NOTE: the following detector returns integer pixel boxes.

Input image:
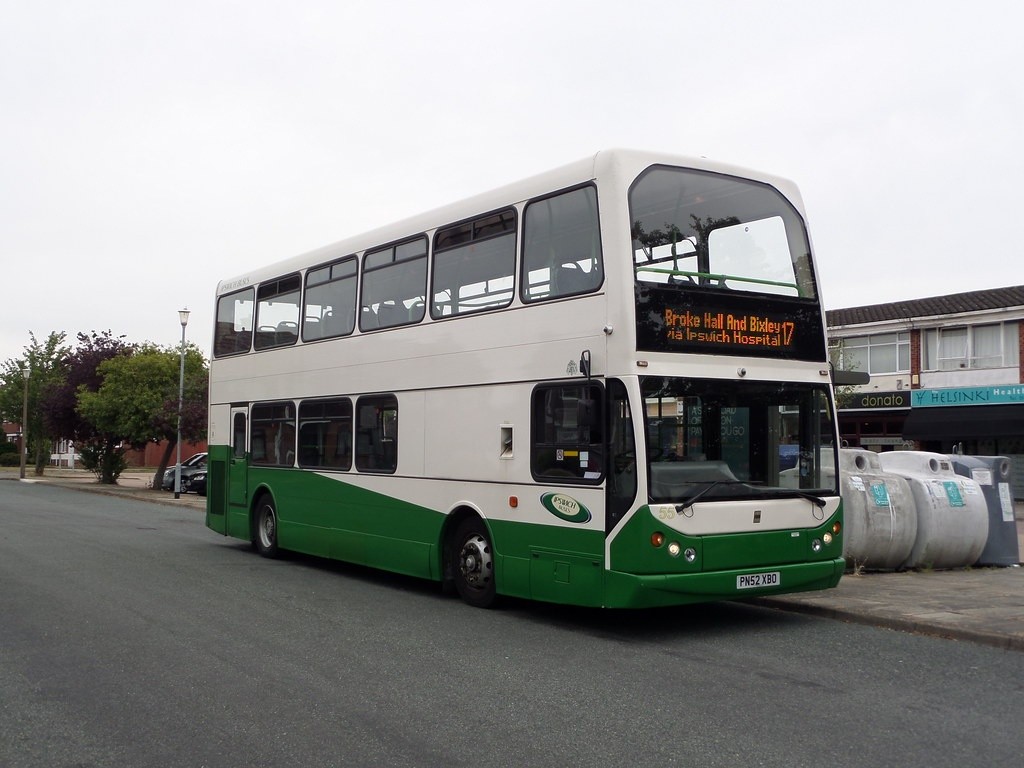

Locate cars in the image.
[176,453,209,496]
[155,452,208,491]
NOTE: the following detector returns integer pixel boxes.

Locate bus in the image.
[206,148,846,614]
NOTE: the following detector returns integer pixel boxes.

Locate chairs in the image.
[218,255,731,357]
